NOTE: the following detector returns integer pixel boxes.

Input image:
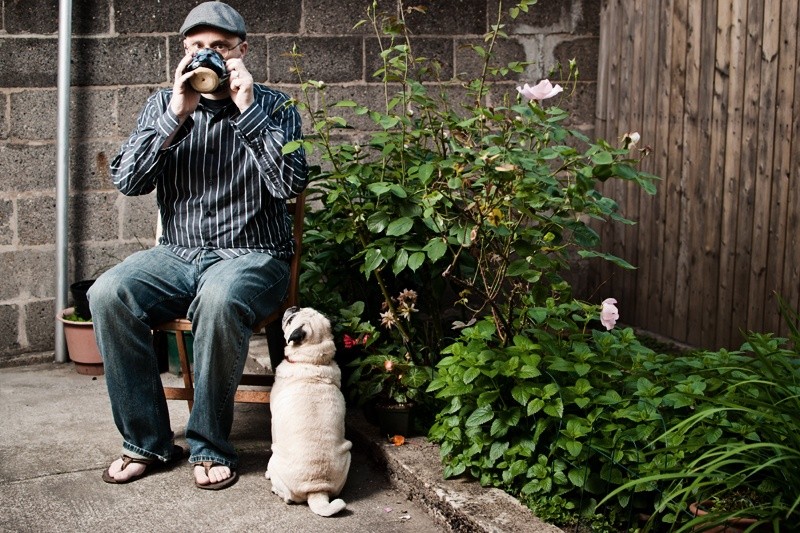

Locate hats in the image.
[180,1,247,41]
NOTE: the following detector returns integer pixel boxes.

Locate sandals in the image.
[192,461,237,490]
[102,432,183,484]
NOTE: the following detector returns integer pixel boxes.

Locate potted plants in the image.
[54,306,104,378]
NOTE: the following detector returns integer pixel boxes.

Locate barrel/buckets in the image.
[70,279,96,320]
[166,330,194,374]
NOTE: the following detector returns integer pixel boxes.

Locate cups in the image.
[186,48,231,92]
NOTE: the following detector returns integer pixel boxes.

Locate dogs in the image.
[266,304,353,518]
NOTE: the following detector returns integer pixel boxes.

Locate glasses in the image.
[185,41,244,57]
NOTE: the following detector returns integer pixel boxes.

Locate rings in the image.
[232,70,240,78]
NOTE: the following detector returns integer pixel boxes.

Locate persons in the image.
[86,0,310,491]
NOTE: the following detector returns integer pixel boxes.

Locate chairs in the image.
[151,161,323,420]
